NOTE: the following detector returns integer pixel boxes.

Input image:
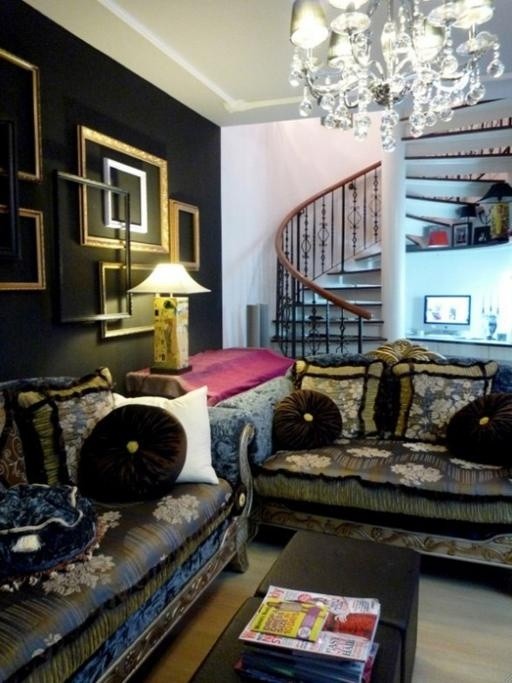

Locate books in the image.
[233,583,382,682]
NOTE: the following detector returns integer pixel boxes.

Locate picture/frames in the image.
[451,222,471,248]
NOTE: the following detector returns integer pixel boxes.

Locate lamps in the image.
[477,179,512,238]
[287,0,505,153]
[127,261,212,375]
